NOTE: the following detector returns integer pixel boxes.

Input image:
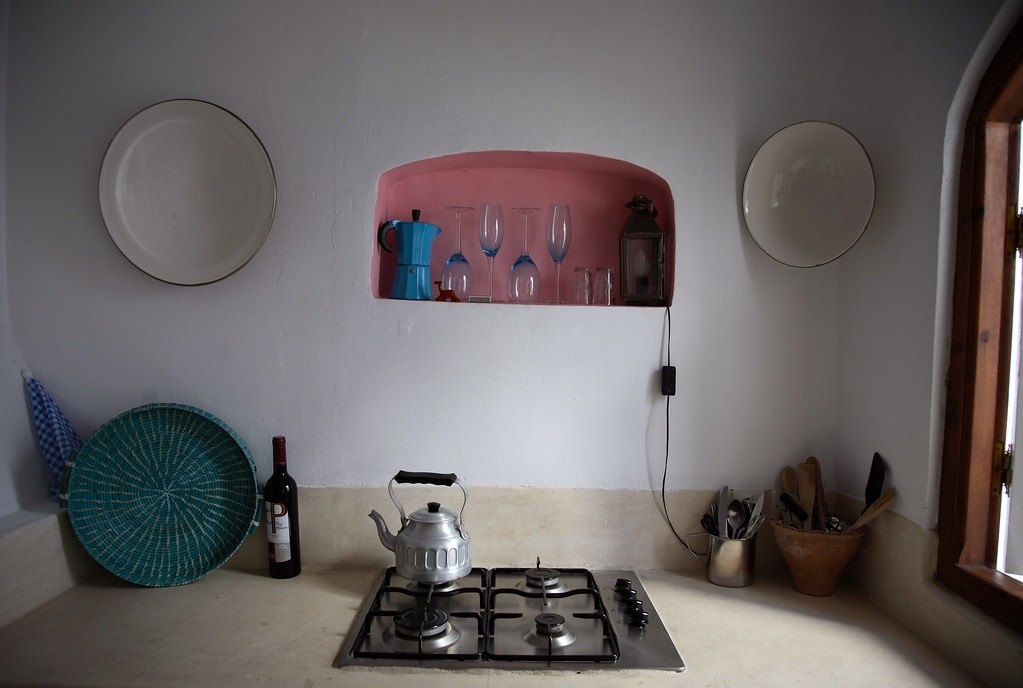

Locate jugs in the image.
[378,208,442,301]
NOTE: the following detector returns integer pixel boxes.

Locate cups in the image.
[686,532,757,588]
[575,267,615,305]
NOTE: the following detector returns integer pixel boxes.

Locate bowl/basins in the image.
[741,121,877,269]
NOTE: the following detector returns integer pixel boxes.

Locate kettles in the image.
[368,470,472,586]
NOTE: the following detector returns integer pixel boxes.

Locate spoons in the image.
[726,499,756,539]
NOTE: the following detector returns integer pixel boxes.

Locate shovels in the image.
[799,462,816,531]
[861,451,885,516]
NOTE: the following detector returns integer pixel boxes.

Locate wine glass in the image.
[441,203,572,304]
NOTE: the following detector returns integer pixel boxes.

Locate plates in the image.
[97,99,279,287]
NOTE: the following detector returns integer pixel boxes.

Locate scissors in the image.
[701,513,718,537]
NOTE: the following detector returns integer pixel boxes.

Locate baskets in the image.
[67,403,265,587]
[768,520,867,596]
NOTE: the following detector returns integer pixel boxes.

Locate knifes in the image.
[740,491,767,540]
[718,485,733,540]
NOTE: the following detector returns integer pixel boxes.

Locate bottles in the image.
[263,436,303,580]
[619,193,666,306]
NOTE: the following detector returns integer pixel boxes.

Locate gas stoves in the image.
[330,557,686,673]
